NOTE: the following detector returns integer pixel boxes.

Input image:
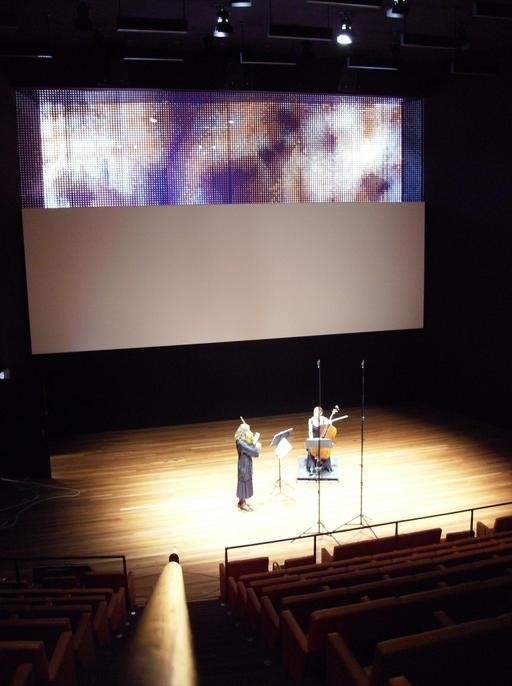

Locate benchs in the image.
[0,565,135,686]
[220,515,512,686]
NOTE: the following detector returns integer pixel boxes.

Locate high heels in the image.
[238,503,253,512]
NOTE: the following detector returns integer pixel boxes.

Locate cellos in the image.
[308,404,340,460]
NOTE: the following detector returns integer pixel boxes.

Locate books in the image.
[275,438,293,459]
[305,438,333,448]
[270,427,294,447]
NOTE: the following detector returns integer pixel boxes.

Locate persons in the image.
[304,406,334,476]
[234,423,261,513]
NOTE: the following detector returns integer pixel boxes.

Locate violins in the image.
[238,416,255,445]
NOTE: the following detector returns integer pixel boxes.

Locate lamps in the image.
[169,553,179,563]
[336,5,353,45]
[213,0,233,37]
[386,0,407,18]
